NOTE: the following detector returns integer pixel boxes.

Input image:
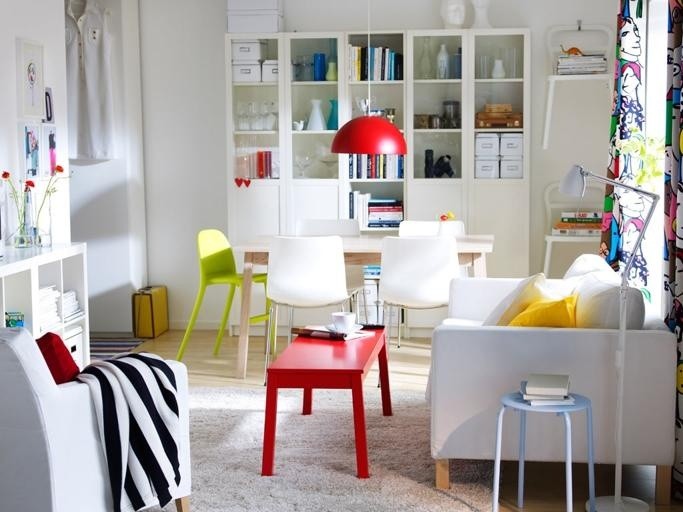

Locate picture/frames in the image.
[13,34,46,123]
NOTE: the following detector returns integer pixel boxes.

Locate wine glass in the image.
[295,145,338,179]
[356,97,396,126]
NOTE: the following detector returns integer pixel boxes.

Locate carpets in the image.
[89,336,145,365]
[138,385,501,511]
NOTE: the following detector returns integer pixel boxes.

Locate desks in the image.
[256,325,393,480]
[235,233,496,383]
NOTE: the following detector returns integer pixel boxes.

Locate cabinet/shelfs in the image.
[223,31,348,341]
[0,241,91,373]
[408,26,533,344]
[347,28,405,326]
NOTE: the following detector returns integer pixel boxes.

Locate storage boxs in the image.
[475,131,522,177]
[226,13,281,33]
[229,36,279,85]
[226,0,282,15]
[60,326,84,371]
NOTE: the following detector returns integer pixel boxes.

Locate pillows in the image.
[497,273,545,326]
[508,289,577,327]
[34,332,79,383]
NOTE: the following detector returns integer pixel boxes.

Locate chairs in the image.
[394,219,472,348]
[263,234,363,387]
[424,252,677,506]
[173,227,275,363]
[377,234,457,386]
[0,325,193,511]
[296,221,367,325]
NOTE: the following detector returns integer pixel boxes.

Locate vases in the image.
[303,98,327,130]
[324,98,339,131]
[11,227,39,247]
[434,42,450,80]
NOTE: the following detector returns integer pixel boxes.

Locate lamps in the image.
[558,163,659,509]
[327,2,408,155]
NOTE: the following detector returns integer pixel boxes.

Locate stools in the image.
[490,392,597,511]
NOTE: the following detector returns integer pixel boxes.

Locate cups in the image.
[479,45,519,80]
[332,311,355,328]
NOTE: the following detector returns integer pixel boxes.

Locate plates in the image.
[326,324,363,334]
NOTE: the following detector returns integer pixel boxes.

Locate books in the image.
[551,209,602,236]
[39,284,83,330]
[349,154,403,229]
[557,55,608,75]
[290,325,375,342]
[348,43,401,80]
[520,373,575,407]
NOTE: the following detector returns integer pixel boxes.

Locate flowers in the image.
[1,163,70,239]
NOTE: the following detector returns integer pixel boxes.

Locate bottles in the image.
[291,54,337,130]
[235,100,279,178]
[439,0,466,29]
[430,44,461,128]
[425,149,433,178]
